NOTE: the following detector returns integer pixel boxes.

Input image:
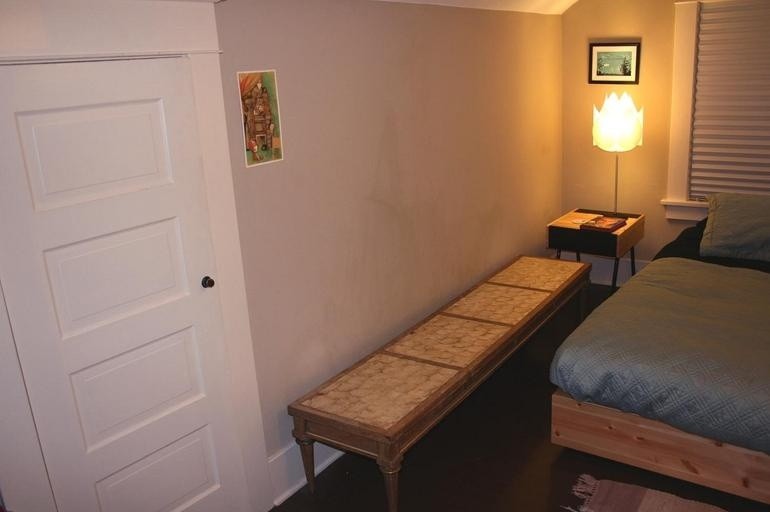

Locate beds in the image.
[550,216,770,505]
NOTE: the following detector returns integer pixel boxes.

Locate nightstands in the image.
[547,208,646,293]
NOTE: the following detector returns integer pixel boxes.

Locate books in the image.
[579,215,628,234]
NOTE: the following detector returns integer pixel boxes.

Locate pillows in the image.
[701,192,770,262]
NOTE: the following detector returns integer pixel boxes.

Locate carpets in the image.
[559,474,728,512]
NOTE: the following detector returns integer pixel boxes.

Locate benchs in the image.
[287,255,592,512]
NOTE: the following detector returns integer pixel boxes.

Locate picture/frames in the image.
[588,41,641,84]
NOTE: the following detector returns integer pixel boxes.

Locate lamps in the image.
[593,91,644,213]
